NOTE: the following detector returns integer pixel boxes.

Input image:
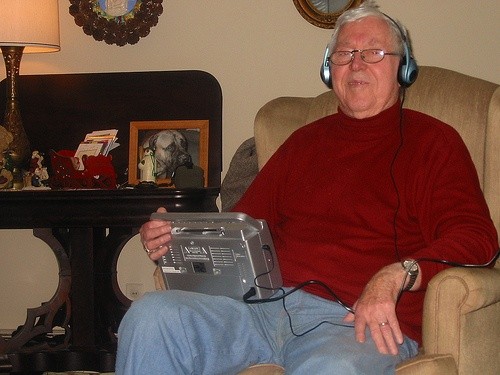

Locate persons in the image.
[115,6,499,375]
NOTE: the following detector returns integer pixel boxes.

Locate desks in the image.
[0,70,223,375]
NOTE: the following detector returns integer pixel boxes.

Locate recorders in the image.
[149,212,283,301]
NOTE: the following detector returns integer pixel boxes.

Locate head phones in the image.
[320,15,419,90]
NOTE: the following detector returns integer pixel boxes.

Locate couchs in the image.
[153,66,500,375]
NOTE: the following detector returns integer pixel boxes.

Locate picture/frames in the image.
[128,120,209,188]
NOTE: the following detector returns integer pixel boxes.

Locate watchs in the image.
[400,258,418,292]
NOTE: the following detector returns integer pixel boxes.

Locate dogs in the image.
[140,129,192,180]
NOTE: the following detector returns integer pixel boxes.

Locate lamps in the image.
[0,0,60,189]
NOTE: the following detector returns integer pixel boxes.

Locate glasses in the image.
[328,49,402,66]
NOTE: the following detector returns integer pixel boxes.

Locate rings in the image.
[379,322,388,326]
[143,243,152,253]
